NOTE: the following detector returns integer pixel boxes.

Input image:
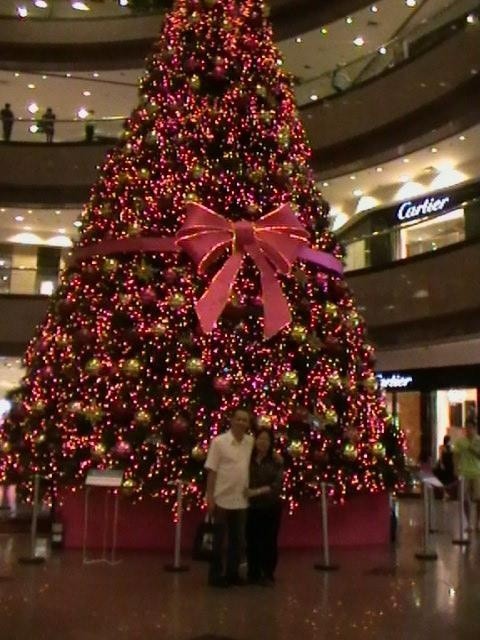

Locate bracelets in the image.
[257,487,261,496]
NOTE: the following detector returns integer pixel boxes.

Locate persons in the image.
[242,426,286,585]
[41,106,56,145]
[433,435,456,501]
[0,103,16,142]
[84,108,97,145]
[202,407,286,587]
[454,533,480,640]
[451,418,480,534]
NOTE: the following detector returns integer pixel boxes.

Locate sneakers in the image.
[209,572,250,589]
